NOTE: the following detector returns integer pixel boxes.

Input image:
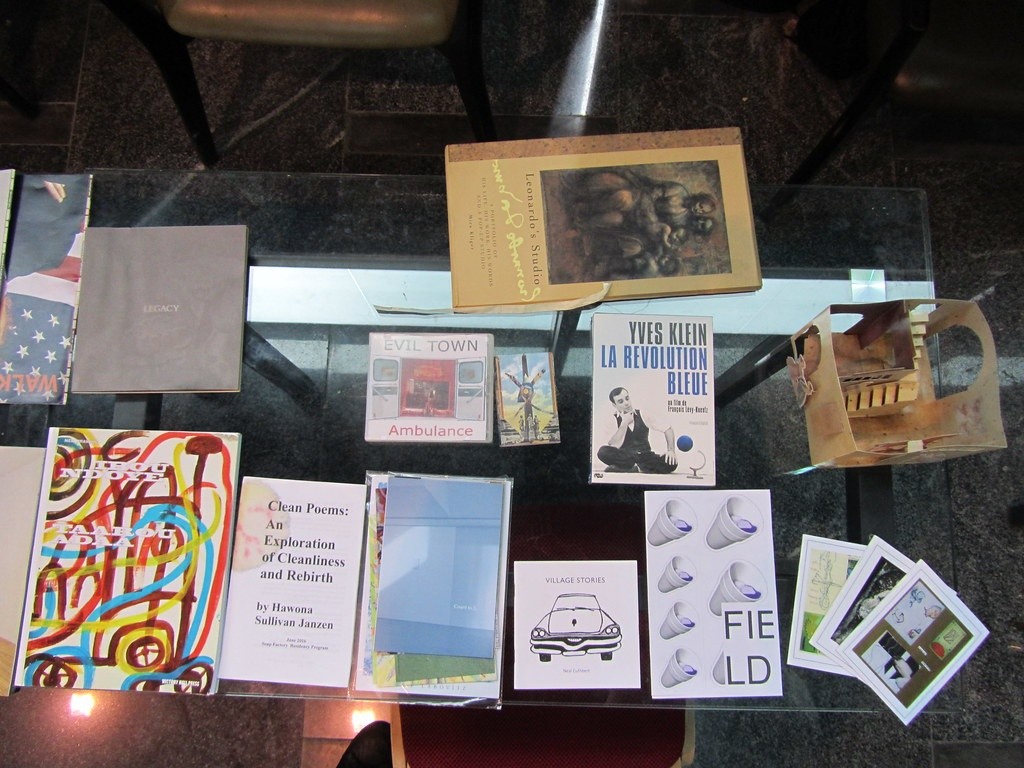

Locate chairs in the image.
[100,0,498,170]
[335,503,695,768]
[759,0,1024,224]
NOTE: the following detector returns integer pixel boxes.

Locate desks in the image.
[0,176,971,720]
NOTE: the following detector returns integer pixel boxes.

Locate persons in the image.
[597,387,678,474]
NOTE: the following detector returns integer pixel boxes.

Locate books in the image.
[786,534,990,726]
[0,426,513,707]
[0,169,248,407]
[444,125,762,315]
[587,313,715,488]
[365,333,561,447]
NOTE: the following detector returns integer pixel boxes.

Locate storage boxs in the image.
[789,298,1009,471]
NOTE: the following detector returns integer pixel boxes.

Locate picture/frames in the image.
[835,558,992,726]
[784,534,867,679]
[807,534,917,687]
